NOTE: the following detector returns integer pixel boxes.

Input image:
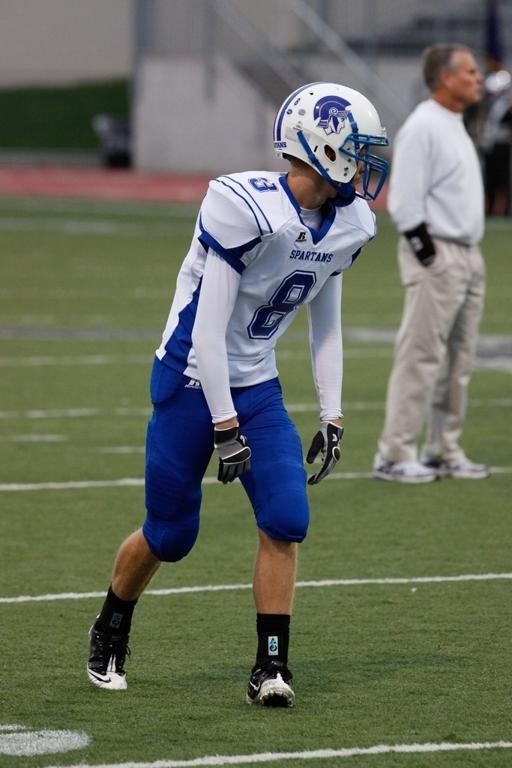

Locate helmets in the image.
[272,81,382,184]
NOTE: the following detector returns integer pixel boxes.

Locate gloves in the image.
[214,424,252,484]
[305,422,344,485]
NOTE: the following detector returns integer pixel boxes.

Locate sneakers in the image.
[246,665,296,707]
[87,613,128,690]
[372,450,438,483]
[422,446,489,479]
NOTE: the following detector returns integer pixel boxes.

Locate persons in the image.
[86,82,390,706]
[369,41,489,486]
